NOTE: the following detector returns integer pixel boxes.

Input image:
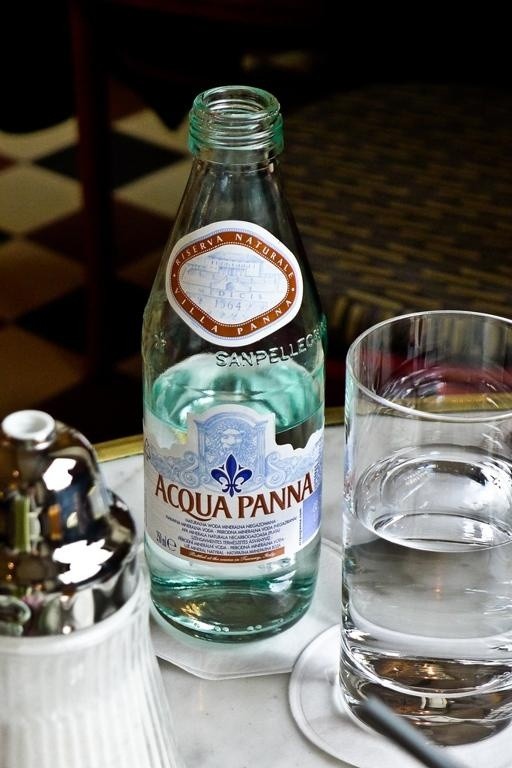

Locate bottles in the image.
[140,81,327,642]
[1,401,184,767]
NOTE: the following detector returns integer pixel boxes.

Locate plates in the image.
[290,620,510,768]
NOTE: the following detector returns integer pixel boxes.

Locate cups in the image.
[337,306,512,744]
